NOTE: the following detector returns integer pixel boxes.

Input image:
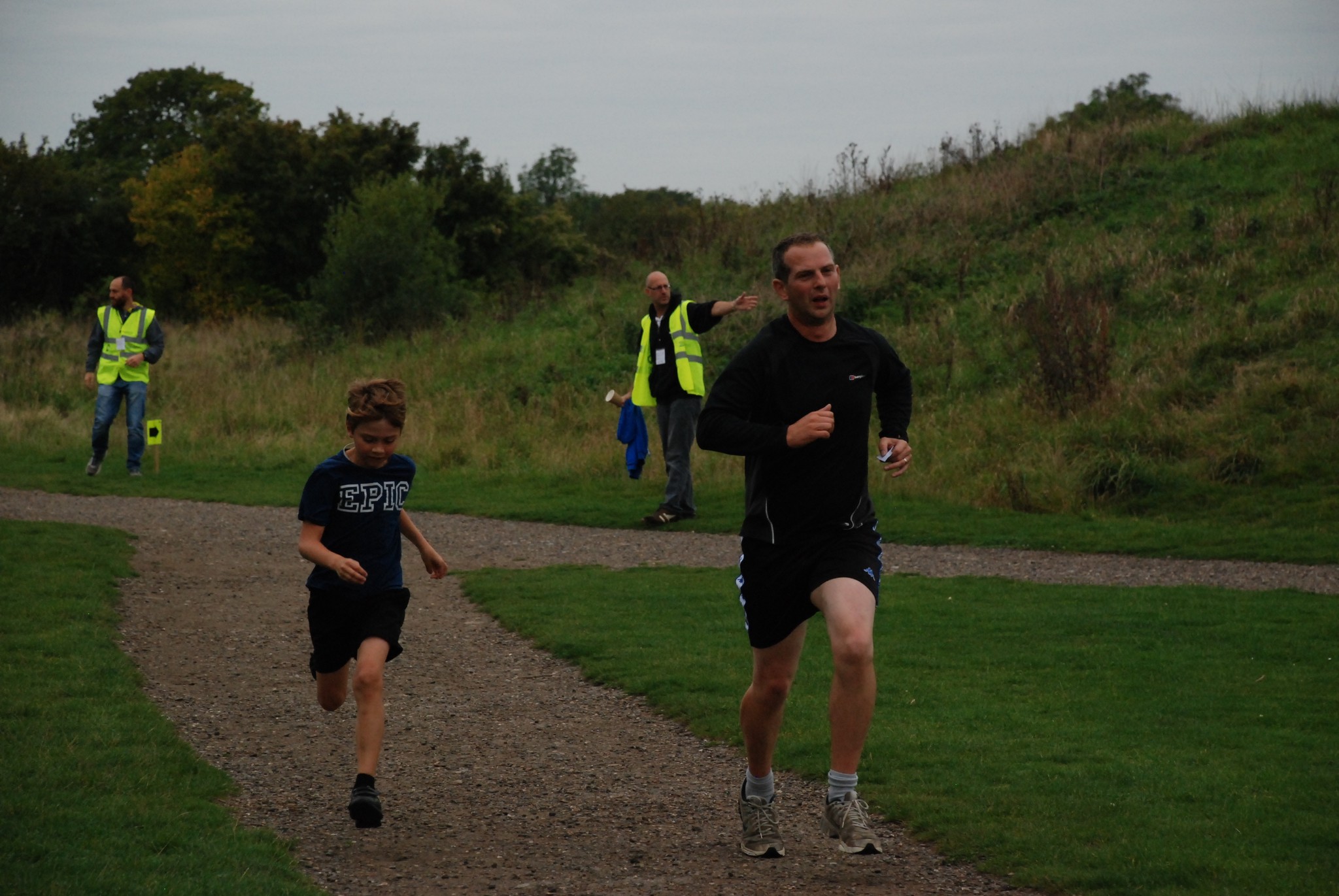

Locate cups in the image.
[605,389,624,407]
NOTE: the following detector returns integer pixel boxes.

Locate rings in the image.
[904,458,907,464]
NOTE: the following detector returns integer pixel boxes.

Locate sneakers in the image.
[309,652,316,679]
[738,776,785,857]
[86,458,101,475]
[130,471,141,477]
[348,786,383,828]
[820,790,882,855]
[644,509,691,525]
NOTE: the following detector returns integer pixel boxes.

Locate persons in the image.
[296,377,449,829]
[695,232,913,859]
[614,271,758,524]
[85,277,166,476]
